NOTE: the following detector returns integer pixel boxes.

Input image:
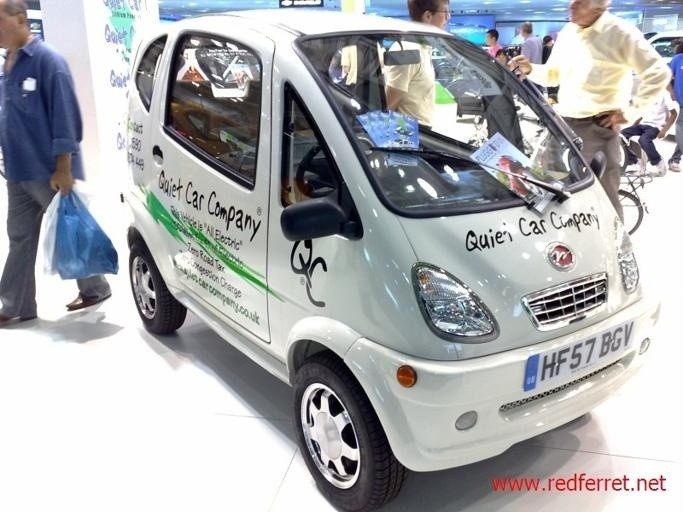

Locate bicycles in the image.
[619,175,653,235]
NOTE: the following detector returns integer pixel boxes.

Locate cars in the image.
[120,7,661,512]
[646,31,683,63]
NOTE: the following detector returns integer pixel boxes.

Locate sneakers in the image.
[669,162,680,172]
[658,160,667,176]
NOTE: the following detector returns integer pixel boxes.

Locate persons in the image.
[0,0,112,326]
[325,0,683,225]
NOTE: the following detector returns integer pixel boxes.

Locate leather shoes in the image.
[0,312,37,322]
[65,294,95,308]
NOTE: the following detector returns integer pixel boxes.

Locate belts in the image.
[576,116,599,122]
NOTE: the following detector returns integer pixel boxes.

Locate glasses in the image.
[423,10,448,20]
